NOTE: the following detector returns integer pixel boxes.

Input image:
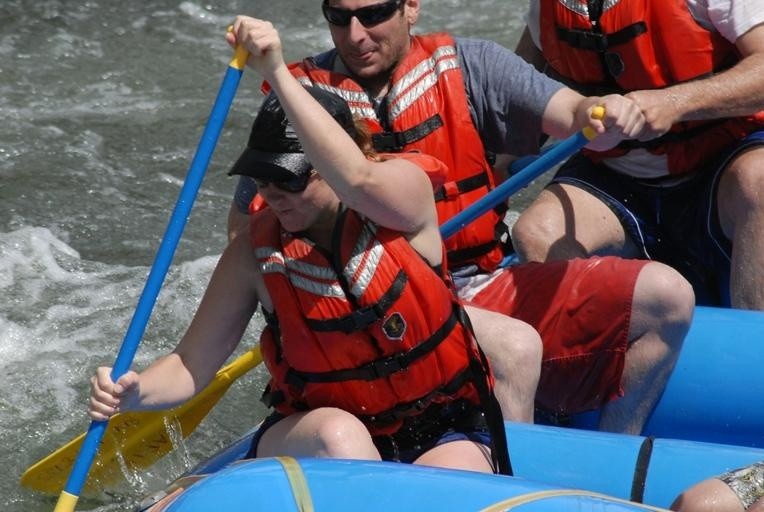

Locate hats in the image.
[227,88,353,178]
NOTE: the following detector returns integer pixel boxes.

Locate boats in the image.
[126,304,762,512]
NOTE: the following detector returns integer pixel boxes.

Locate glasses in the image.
[322,1,409,28]
[249,177,309,193]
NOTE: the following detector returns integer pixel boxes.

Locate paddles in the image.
[19,106,604,494]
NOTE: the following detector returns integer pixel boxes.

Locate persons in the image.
[86,16,507,474]
[669,460,764,512]
[228,0,696,437]
[514,0,764,311]
